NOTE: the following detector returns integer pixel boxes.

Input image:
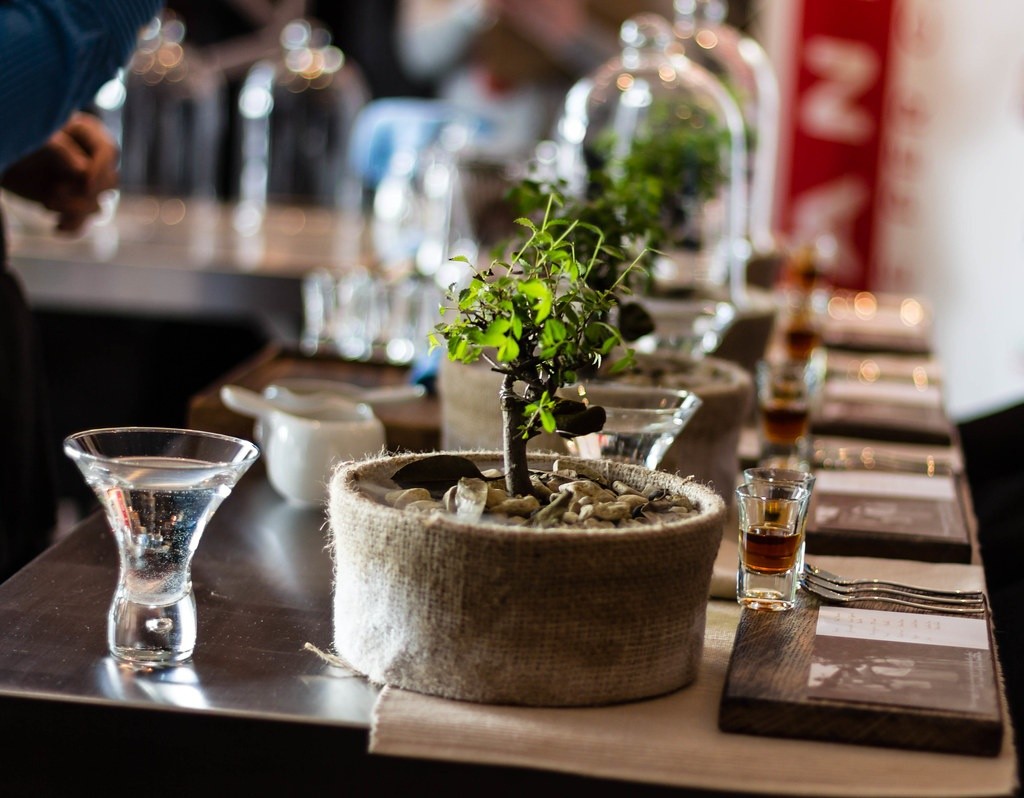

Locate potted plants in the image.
[439,117,755,497]
[326,181,728,708]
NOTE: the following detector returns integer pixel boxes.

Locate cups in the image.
[257,379,426,459]
[757,369,812,463]
[555,384,701,470]
[225,387,385,505]
[60,425,260,665]
[736,483,810,611]
[631,301,733,360]
[745,468,816,590]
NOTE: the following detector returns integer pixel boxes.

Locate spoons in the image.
[801,571,983,604]
[804,562,982,595]
[800,579,985,613]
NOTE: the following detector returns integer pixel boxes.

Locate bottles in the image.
[92,12,219,269]
[243,21,361,275]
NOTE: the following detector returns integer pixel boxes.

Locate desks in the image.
[0,290,1018,798]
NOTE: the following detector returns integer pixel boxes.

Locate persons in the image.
[156,0,754,251]
[0,0,165,586]
